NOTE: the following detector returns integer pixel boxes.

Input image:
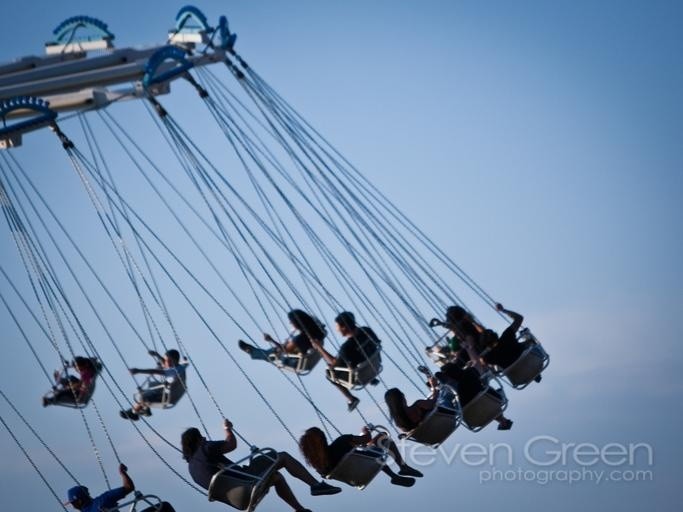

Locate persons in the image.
[120,350,188,420]
[63,464,176,512]
[238,309,381,412]
[42,357,101,407]
[181,419,341,512]
[300,427,423,487]
[384,304,543,445]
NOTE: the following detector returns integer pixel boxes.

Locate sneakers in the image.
[42,370,62,408]
[311,480,342,496]
[238,340,252,354]
[497,419,512,429]
[390,465,424,487]
[119,407,152,421]
[347,379,381,413]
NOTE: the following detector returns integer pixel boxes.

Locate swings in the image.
[0,50,550,512]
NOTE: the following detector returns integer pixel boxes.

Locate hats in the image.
[64,486,89,507]
